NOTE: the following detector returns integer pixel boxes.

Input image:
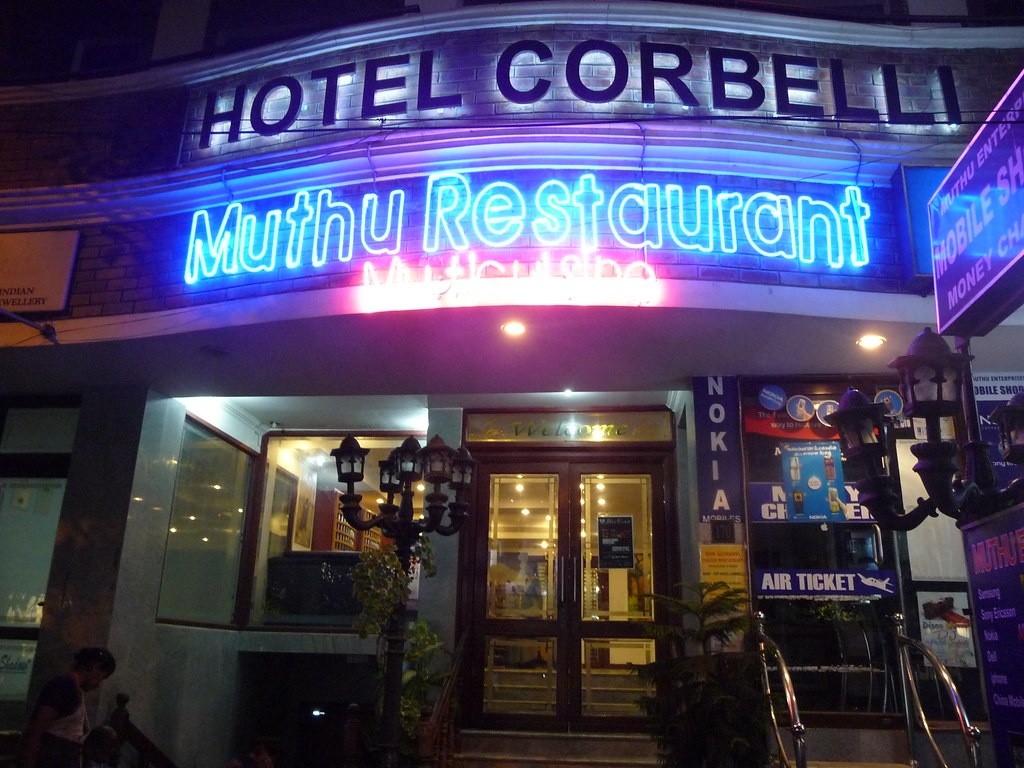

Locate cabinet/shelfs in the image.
[312,490,381,551]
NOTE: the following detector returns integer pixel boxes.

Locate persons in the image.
[522,578,543,610]
[18,646,115,768]
[631,554,645,611]
[82,725,151,768]
[299,498,309,531]
